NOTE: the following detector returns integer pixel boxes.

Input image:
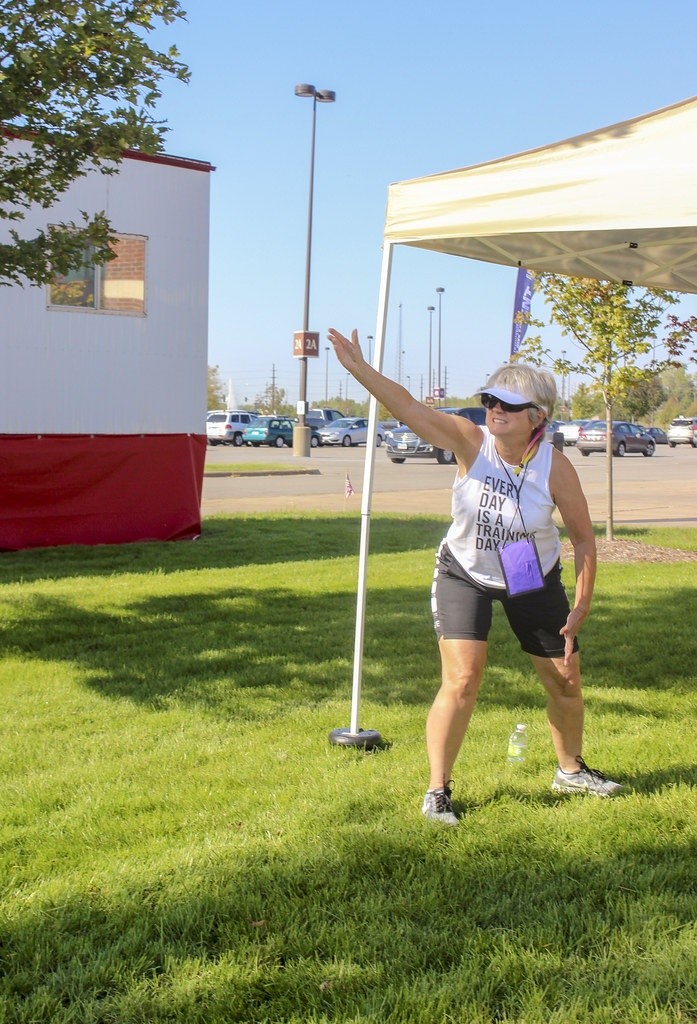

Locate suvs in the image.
[206,413,258,448]
[666,417,697,449]
[207,410,261,415]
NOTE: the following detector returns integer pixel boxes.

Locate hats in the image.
[473,380,549,418]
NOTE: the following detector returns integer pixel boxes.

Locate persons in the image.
[329,327,623,825]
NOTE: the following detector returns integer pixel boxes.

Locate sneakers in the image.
[553,755,624,797]
[422,780,460,826]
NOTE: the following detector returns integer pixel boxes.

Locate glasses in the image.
[481,393,547,423]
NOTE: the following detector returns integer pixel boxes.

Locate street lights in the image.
[427,306,434,406]
[292,82,338,457]
[436,287,445,408]
[368,335,373,366]
[325,347,330,408]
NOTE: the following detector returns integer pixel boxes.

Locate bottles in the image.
[506,723,528,766]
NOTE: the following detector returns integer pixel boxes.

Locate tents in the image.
[350,94,697,739]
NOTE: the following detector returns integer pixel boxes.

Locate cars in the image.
[643,427,669,445]
[576,420,656,457]
[558,420,589,447]
[544,421,564,443]
[241,417,297,448]
[306,408,403,447]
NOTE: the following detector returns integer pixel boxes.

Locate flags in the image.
[511,268,544,356]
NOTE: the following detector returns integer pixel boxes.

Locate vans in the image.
[386,407,486,465]
